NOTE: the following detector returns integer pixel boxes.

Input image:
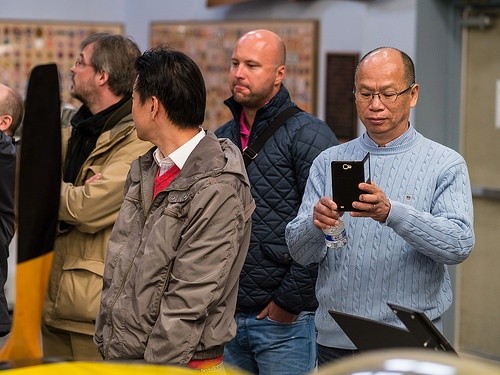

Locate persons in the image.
[41,31,156,364]
[212,28,339,375]
[0,84,24,337]
[285,46,475,370]
[92,44,257,374]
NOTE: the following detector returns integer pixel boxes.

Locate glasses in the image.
[75,58,92,68]
[352,84,414,103]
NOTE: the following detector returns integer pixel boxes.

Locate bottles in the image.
[322,219,347,248]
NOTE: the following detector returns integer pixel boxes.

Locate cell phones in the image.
[332,152,371,212]
